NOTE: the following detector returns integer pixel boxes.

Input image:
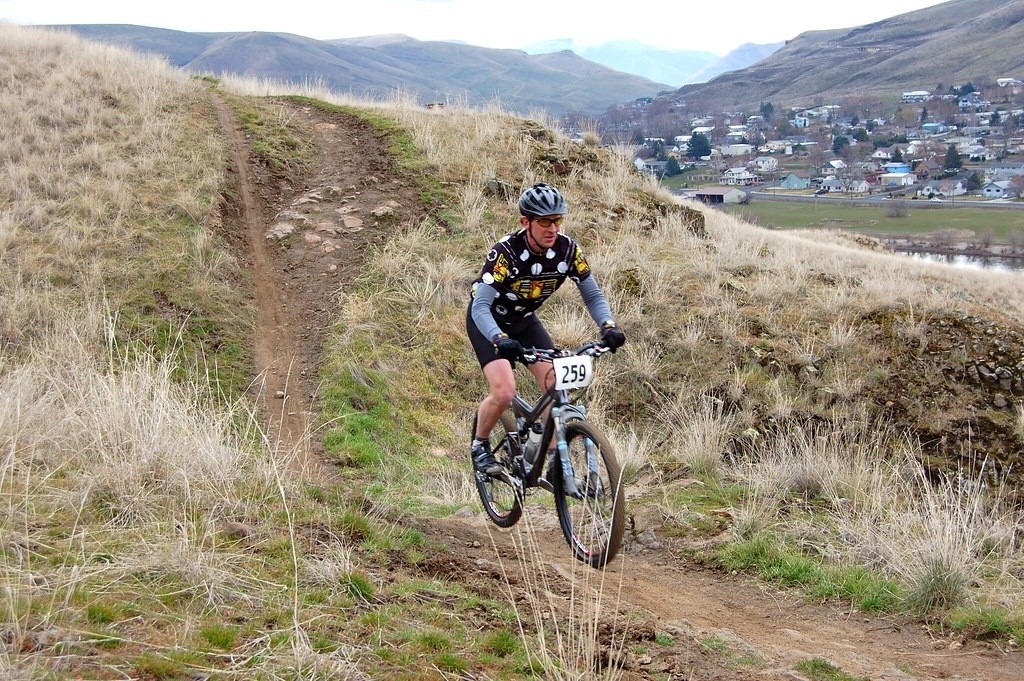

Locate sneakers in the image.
[471,440,502,475]
[546,466,578,494]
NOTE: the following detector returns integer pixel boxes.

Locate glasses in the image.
[533,217,564,227]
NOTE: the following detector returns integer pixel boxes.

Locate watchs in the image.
[601,320,616,328]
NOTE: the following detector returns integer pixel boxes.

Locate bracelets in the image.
[493,333,508,342]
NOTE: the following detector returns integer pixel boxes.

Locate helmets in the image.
[519,183,567,218]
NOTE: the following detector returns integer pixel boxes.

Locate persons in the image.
[889,193,892,197]
[467,183,627,487]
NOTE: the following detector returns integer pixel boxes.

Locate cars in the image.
[1002,193,1012,199]
[815,189,828,195]
[930,198,942,203]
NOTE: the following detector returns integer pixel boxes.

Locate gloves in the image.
[494,339,524,362]
[601,320,625,354]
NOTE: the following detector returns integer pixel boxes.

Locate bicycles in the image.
[471,338,626,569]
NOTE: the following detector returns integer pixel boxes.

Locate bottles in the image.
[523,422,544,466]
